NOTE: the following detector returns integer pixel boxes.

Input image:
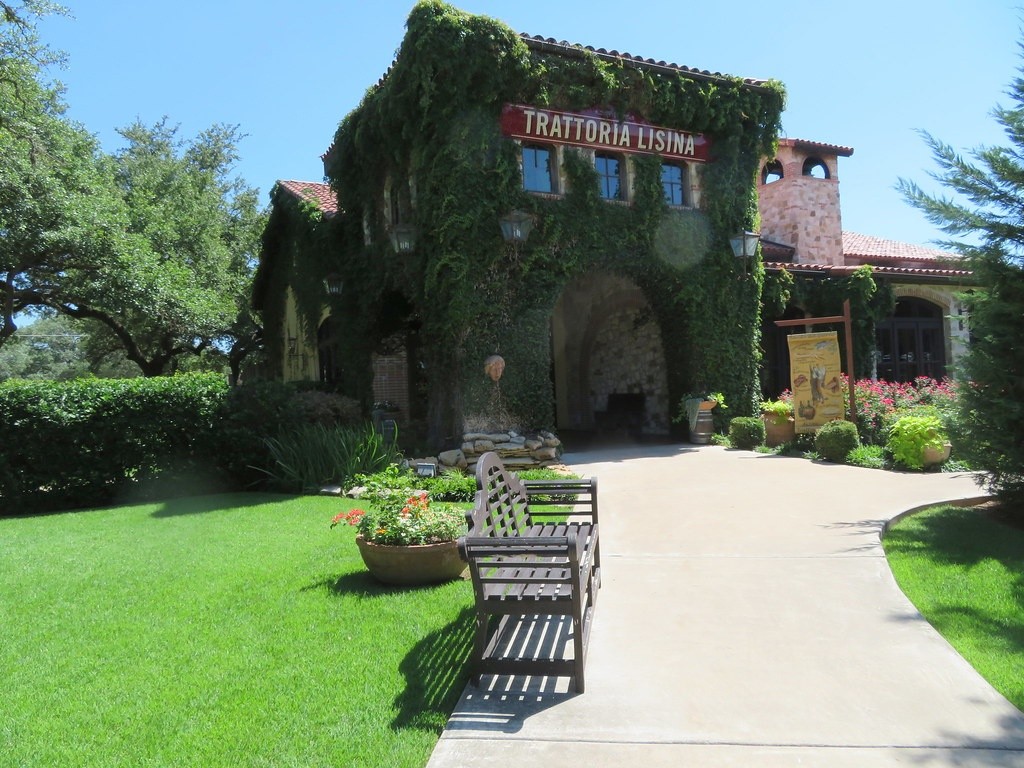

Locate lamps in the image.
[416,463,435,478]
[497,207,534,261]
[728,225,759,278]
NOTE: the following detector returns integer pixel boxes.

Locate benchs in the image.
[457,452,602,693]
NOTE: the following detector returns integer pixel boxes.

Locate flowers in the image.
[331,489,471,546]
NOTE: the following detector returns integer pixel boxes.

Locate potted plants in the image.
[673,392,728,430]
[888,416,952,471]
[727,416,764,449]
[760,400,795,443]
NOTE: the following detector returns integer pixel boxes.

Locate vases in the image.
[355,533,469,586]
